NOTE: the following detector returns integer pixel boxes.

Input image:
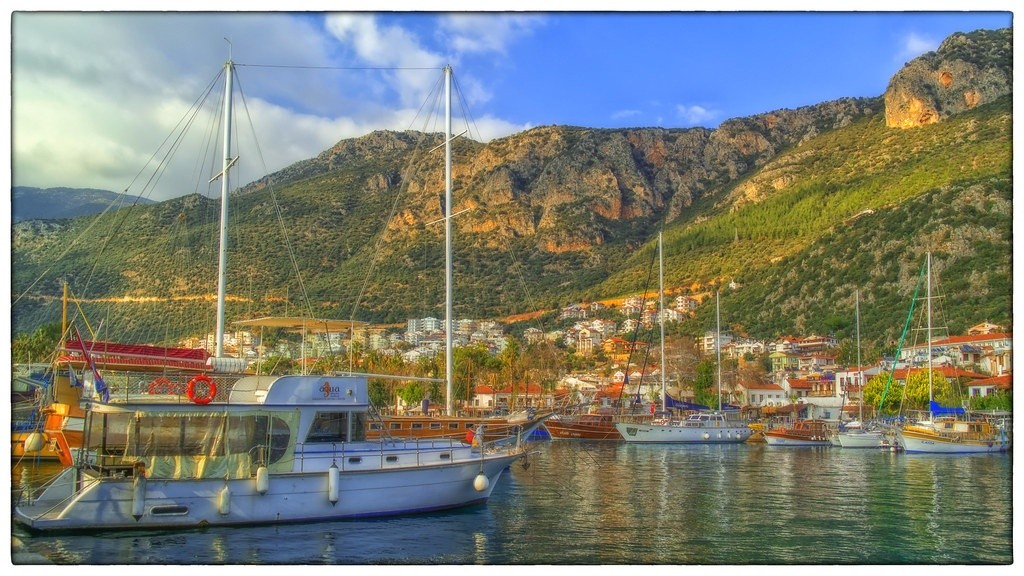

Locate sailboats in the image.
[16,35,562,536]
[540,231,758,442]
[747,247,1012,452]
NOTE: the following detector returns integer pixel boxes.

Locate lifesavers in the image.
[172,383,182,394]
[186,375,216,404]
[148,377,173,396]
